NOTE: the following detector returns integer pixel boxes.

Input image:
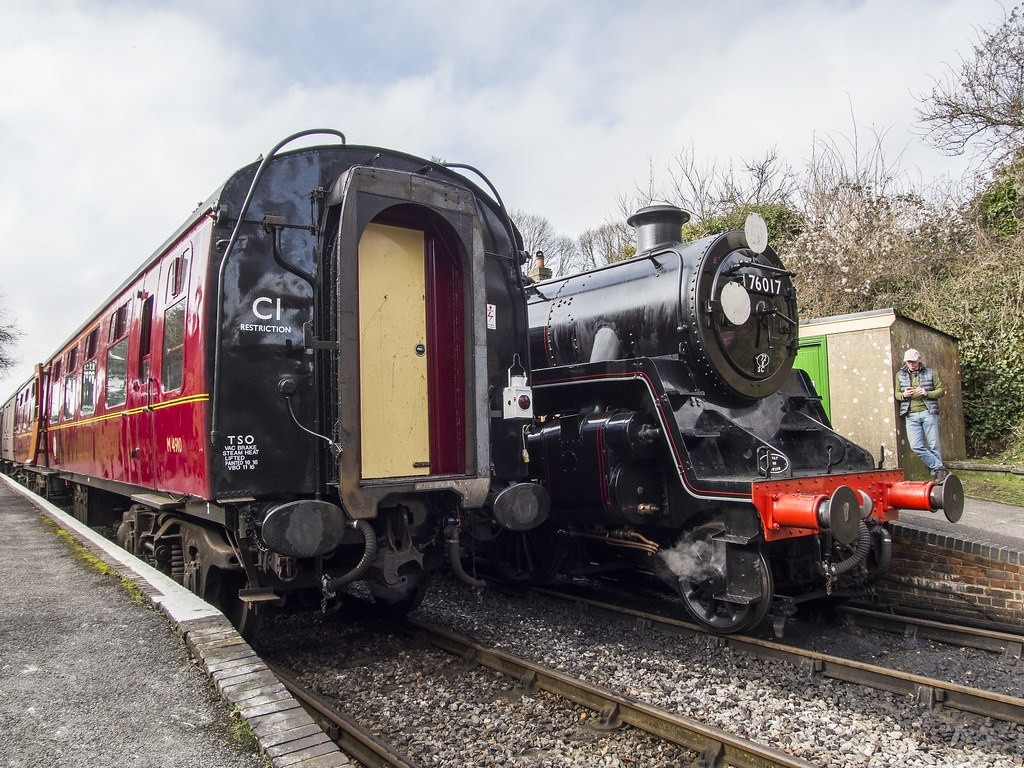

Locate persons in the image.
[895,348,952,485]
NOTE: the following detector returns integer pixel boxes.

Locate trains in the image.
[1,128,551,648]
[524,203,965,634]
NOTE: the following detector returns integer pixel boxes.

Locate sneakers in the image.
[932,466,952,484]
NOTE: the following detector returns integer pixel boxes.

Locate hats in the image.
[903,349,921,361]
